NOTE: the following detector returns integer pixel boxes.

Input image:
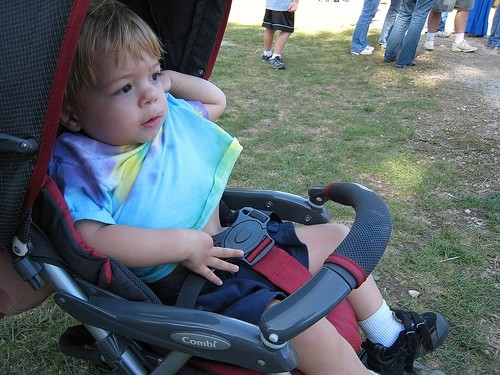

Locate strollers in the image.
[0,1,423,375]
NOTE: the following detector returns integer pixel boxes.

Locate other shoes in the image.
[385,56,398,61]
[379,45,386,50]
[396,61,417,68]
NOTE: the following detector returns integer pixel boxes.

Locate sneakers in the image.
[365,45,375,52]
[423,41,434,51]
[268,56,285,68]
[436,31,451,37]
[362,308,449,375]
[451,40,477,53]
[351,48,372,56]
[262,54,272,62]
[426,34,434,40]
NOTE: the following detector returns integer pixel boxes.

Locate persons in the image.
[262,0,299,68]
[48,0,451,375]
[350,0,500,68]
